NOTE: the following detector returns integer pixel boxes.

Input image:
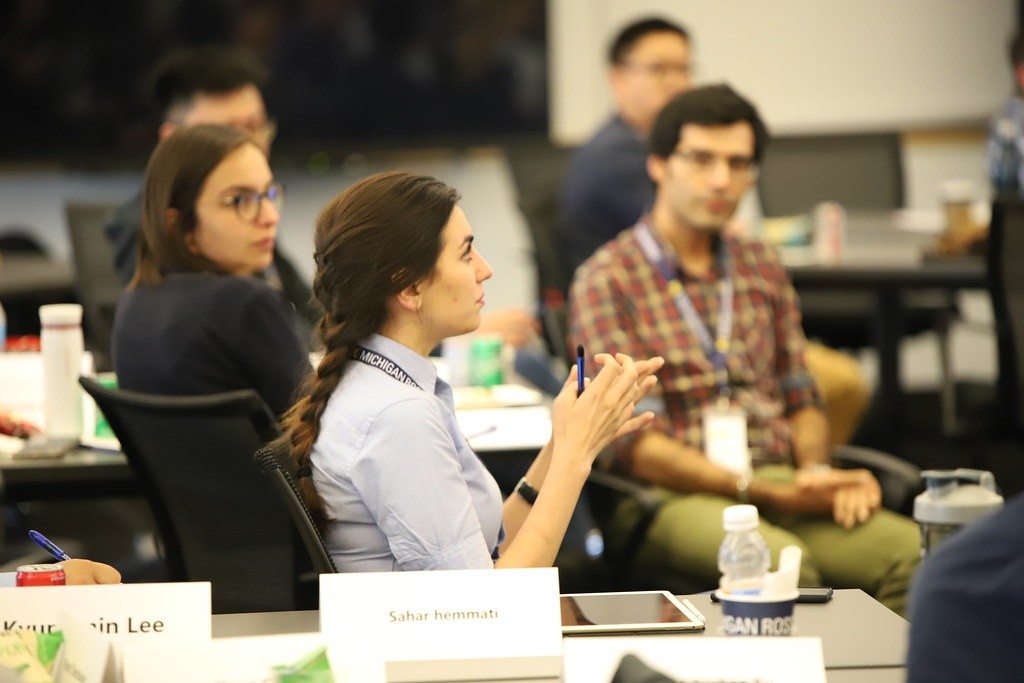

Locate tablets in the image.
[561,590,705,634]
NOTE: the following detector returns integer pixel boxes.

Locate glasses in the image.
[675,149,761,184]
[220,182,287,221]
[251,120,277,148]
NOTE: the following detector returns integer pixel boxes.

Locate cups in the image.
[714,588,800,638]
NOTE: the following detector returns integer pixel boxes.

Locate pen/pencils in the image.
[29,530,70,561]
[577,344,585,397]
[682,599,706,622]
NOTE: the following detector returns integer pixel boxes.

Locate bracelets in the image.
[513,477,540,505]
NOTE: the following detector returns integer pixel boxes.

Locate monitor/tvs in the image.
[1,0,548,181]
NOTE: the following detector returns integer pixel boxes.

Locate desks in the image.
[783,221,992,444]
[11,377,554,455]
[210,584,908,682]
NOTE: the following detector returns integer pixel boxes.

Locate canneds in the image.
[813,201,844,261]
[468,336,505,386]
[16,564,65,587]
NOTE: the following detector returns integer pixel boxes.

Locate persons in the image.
[263,168,667,574]
[536,18,703,302]
[908,1,1024,683]
[107,124,330,534]
[0,559,122,587]
[563,85,928,619]
[102,54,328,355]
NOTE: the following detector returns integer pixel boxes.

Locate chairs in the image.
[78,378,344,612]
[66,204,145,368]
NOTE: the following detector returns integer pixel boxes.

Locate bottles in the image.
[716,505,773,587]
[35,304,87,444]
[912,467,1006,563]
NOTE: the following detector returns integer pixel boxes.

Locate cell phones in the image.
[710,586,833,603]
[13,439,78,460]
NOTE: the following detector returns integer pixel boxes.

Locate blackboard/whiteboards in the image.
[544,0,1024,151]
